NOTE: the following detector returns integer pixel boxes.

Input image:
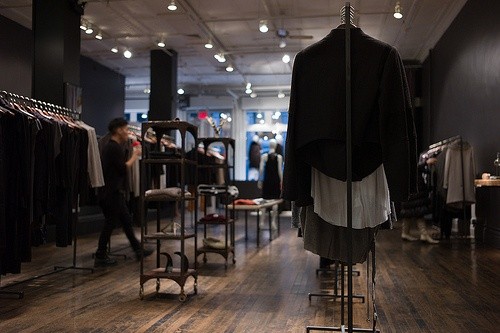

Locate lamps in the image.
[166,0,178,11]
[244,80,285,99]
[156,37,166,48]
[225,63,234,73]
[203,37,214,50]
[393,1,404,20]
[259,19,291,64]
[80,19,134,60]
[213,51,227,63]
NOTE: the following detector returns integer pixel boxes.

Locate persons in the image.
[93,117,156,267]
[257,139,283,214]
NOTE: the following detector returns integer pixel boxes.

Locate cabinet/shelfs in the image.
[138,121,200,302]
[195,135,237,271]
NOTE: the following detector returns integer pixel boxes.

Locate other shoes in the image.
[420,234,440,244]
[401,234,418,242]
[93,258,118,267]
[137,249,154,261]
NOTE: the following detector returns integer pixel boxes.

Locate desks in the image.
[474,178,500,245]
[222,198,284,249]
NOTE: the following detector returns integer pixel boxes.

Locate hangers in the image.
[427,134,464,151]
[0,90,81,128]
[337,5,357,28]
